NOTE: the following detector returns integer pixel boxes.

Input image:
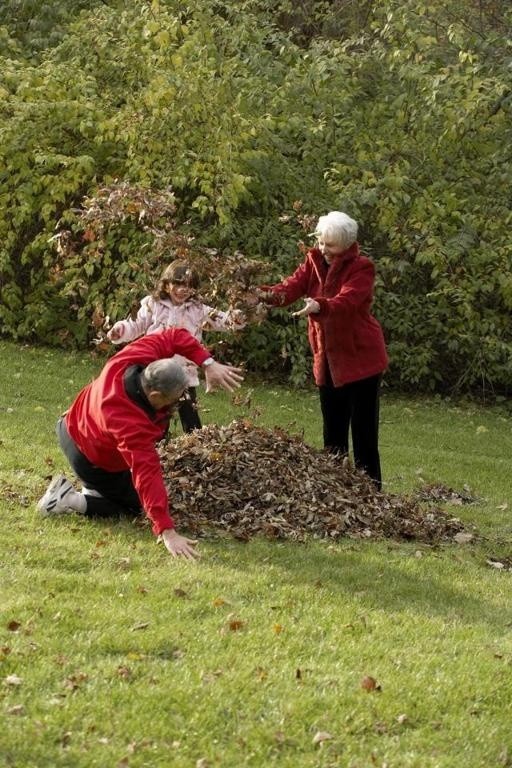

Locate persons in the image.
[104,258,250,433]
[253,210,390,494]
[33,325,247,562]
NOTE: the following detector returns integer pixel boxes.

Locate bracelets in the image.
[200,357,215,369]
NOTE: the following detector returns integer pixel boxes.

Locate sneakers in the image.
[36,472,73,517]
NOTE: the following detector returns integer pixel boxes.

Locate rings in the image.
[228,370,234,376]
[177,550,184,555]
